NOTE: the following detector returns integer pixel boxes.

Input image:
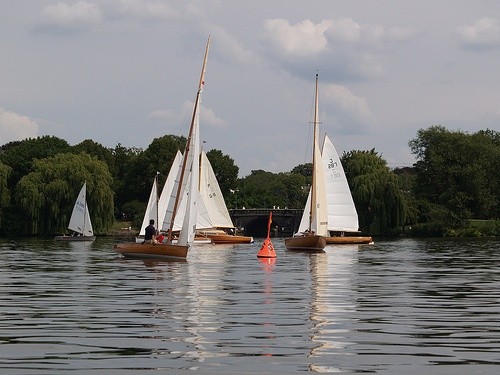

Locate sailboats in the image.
[55,180,96,240]
[284,73,326,251]
[136,142,254,246]
[297,133,372,244]
[114,34,211,259]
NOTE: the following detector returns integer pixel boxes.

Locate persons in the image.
[144,219,163,245]
[243,205,288,210]
[156,232,166,244]
